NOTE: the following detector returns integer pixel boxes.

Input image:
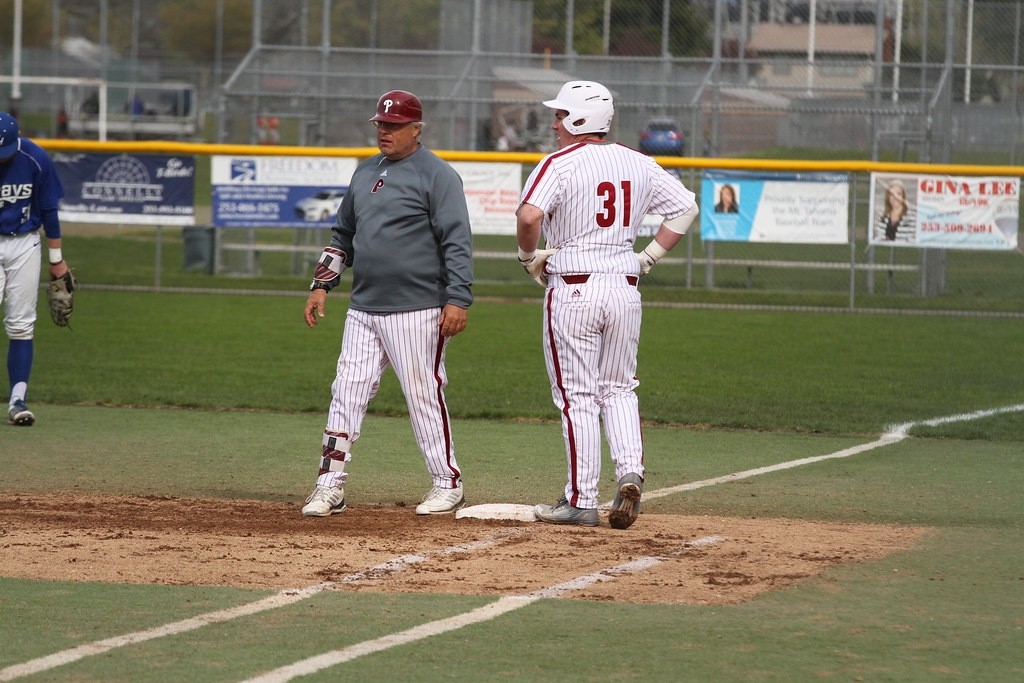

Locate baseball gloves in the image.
[46,269,77,327]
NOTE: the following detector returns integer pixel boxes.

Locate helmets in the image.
[368,90,422,124]
[542,80,613,137]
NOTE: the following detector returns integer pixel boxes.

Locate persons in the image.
[715,184,738,212]
[874,179,916,242]
[302,90,474,518]
[80,90,100,117]
[514,80,699,530]
[124,94,145,118]
[0,112,69,426]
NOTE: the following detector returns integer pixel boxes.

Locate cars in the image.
[639,118,691,156]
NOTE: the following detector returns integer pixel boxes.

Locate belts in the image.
[563,273,639,286]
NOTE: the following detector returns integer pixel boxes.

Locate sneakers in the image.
[535,493,599,526]
[302,484,346,516]
[416,484,467,515]
[8,398,35,425]
[608,472,643,529]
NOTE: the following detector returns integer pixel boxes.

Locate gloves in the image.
[517,245,561,288]
[635,239,669,277]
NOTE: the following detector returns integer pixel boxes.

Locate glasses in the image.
[373,120,415,128]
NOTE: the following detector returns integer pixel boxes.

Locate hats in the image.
[0,111,19,157]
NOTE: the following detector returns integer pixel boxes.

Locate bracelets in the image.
[49,248,62,265]
